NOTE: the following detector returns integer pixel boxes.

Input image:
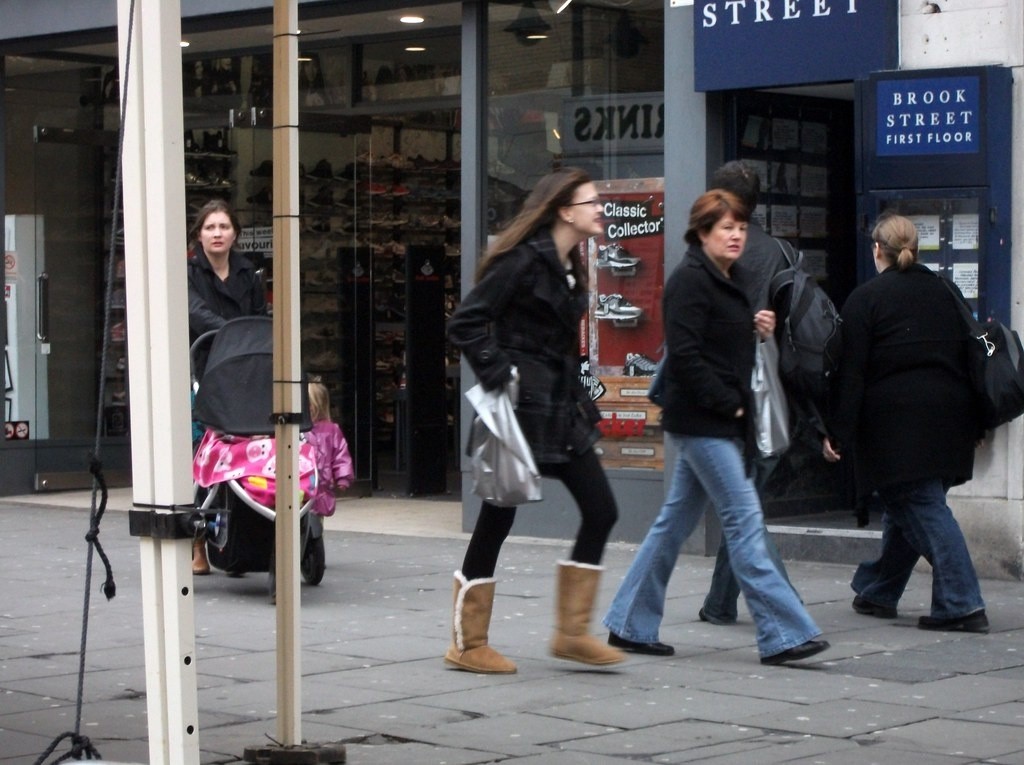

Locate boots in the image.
[447,570,517,675]
[191,538,209,574]
[553,558,628,665]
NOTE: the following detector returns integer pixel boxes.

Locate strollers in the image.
[188,314,331,601]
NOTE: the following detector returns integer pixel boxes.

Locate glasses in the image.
[563,195,603,210]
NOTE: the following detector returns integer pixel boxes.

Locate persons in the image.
[443,165,624,675]
[304,382,353,570]
[698,158,840,626]
[828,214,991,633]
[601,188,832,664]
[187,199,268,577]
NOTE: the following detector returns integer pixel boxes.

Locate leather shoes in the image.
[762,640,831,664]
[608,629,676,655]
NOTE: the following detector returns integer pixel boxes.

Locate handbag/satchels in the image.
[749,330,791,455]
[465,366,543,506]
[938,273,1023,436]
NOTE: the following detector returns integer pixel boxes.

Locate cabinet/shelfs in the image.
[99,108,462,454]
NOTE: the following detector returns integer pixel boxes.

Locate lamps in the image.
[548,0,573,15]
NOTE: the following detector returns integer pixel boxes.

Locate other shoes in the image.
[918,610,990,631]
[852,597,899,618]
[698,608,708,622]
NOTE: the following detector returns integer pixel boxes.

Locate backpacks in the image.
[763,238,842,372]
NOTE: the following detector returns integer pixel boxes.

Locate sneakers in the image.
[72,43,519,446]
[594,292,643,320]
[622,352,659,378]
[597,242,644,267]
